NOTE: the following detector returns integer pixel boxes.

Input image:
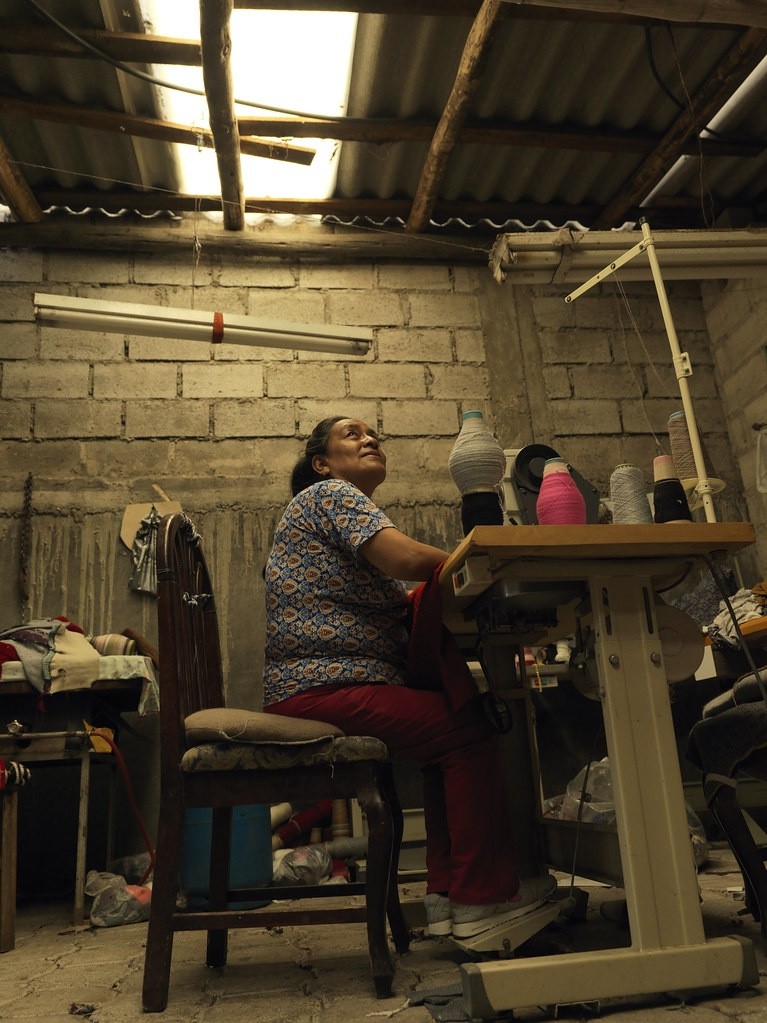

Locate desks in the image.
[400,516,757,1020]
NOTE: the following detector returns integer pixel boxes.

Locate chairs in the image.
[139,512,407,1015]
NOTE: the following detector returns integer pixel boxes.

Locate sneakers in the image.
[424,893,452,937]
[451,875,558,939]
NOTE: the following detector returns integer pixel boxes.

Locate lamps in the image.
[488,226,766,286]
[29,288,375,359]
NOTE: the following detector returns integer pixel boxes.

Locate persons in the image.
[262,417,557,937]
